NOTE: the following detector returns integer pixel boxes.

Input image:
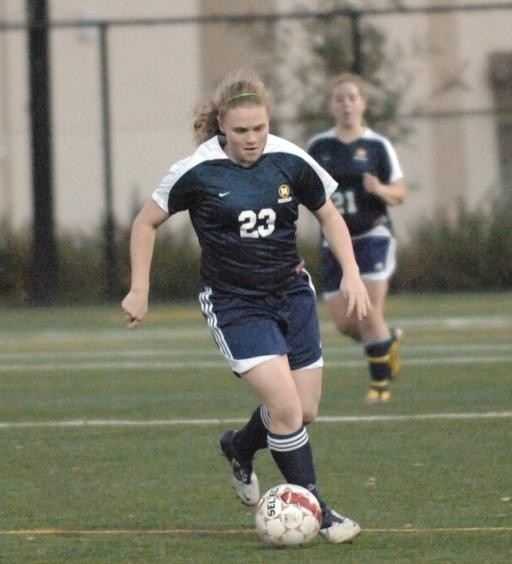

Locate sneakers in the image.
[386,327,404,379]
[218,423,264,507]
[365,376,394,404]
[316,504,361,544]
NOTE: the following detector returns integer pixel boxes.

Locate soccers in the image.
[255,483,322,547]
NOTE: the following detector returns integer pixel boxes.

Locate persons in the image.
[121,74,372,542]
[302,71,406,401]
